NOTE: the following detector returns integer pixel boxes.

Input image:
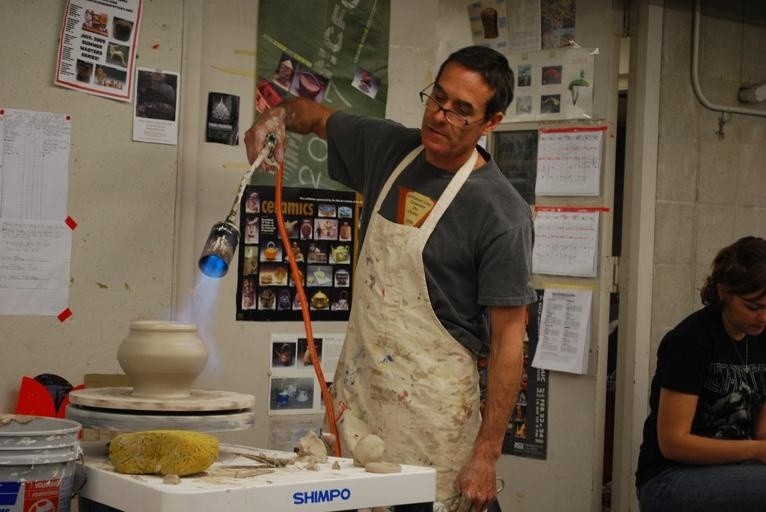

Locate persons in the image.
[324,221,333,237]
[331,222,337,237]
[243,45,536,512]
[634,236,765,511]
[315,223,323,238]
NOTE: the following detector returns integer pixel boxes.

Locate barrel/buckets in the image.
[0,416,88,512]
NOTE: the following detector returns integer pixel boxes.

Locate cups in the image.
[275,384,309,407]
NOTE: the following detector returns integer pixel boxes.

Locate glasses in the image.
[419,82,486,129]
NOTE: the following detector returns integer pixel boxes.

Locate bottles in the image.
[302,346,319,366]
[117,320,209,399]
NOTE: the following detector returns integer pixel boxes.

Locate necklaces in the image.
[725,324,752,375]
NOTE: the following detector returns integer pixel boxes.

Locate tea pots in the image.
[274,344,292,366]
[243,216,352,312]
